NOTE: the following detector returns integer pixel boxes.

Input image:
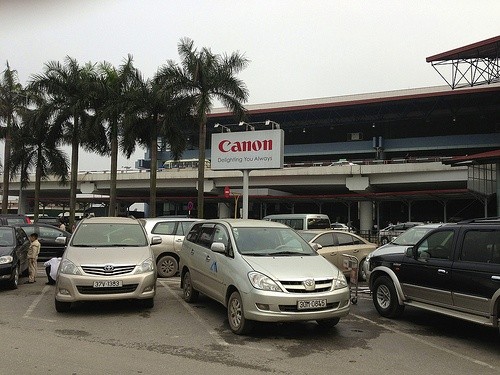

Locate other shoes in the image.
[23,280,36,283]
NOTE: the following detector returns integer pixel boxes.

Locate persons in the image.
[43,250,62,285]
[24,233,41,284]
[59,220,66,231]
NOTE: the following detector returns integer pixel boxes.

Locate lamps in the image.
[214,120,280,132]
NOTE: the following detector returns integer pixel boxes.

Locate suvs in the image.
[362,218,500,337]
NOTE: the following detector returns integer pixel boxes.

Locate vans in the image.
[58,212,95,229]
[261,214,332,232]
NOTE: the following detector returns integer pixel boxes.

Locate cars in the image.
[378,221,425,244]
[330,222,357,234]
[176,218,351,336]
[283,228,378,283]
[54,212,163,314]
[138,217,205,278]
[0,213,61,228]
[14,223,71,262]
[0,225,33,289]
